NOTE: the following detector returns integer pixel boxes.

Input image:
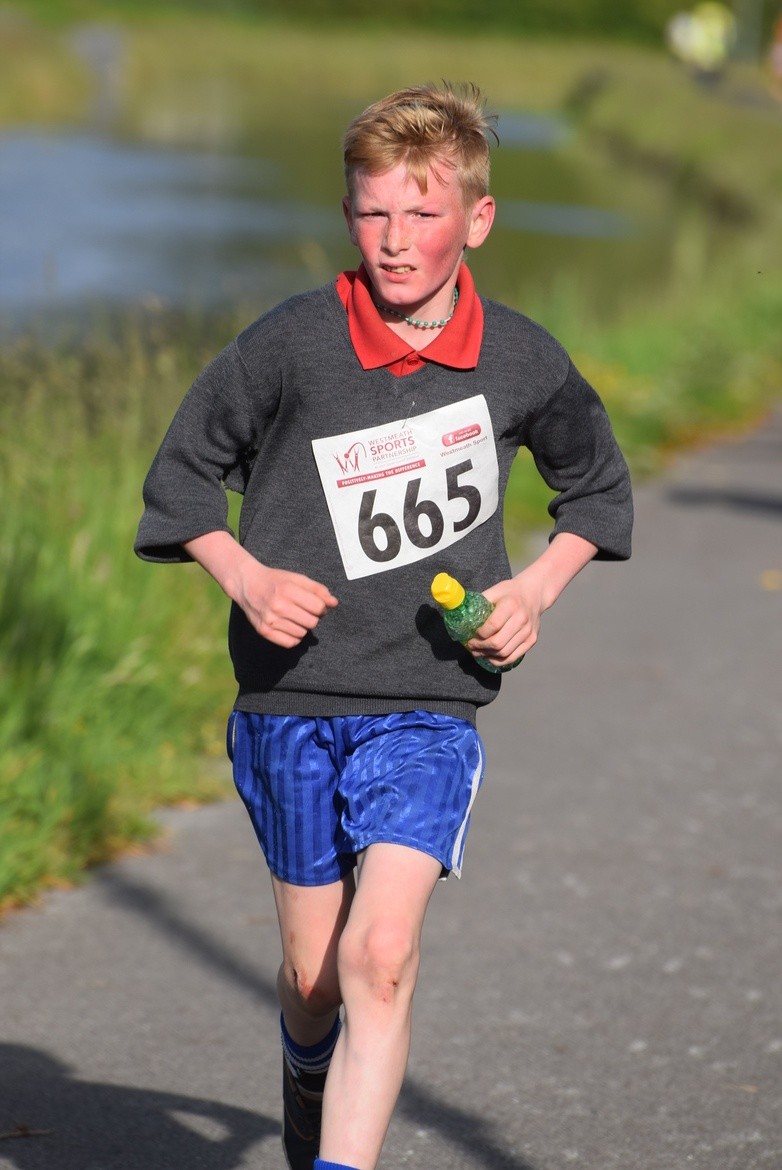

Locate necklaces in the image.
[371,287,459,327]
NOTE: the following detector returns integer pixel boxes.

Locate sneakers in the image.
[281,1045,328,1170]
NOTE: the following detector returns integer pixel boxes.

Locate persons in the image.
[133,88,635,1170]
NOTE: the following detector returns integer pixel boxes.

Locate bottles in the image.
[432,573,525,672]
